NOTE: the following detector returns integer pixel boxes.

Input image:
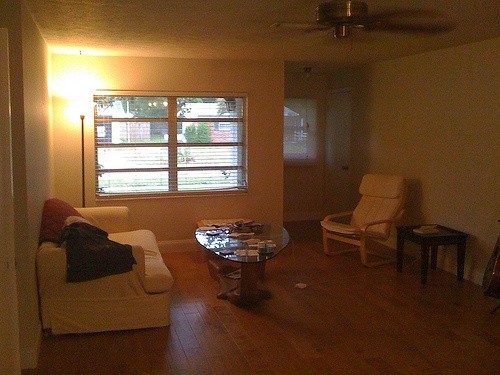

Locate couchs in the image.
[36,198,174,337]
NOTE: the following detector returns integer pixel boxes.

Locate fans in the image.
[266,0,458,43]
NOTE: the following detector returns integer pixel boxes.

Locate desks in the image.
[396,224,469,285]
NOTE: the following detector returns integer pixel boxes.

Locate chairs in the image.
[320,173,409,268]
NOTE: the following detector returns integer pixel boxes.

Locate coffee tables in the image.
[195,220,290,310]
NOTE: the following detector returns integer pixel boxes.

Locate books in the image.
[413,224,439,234]
